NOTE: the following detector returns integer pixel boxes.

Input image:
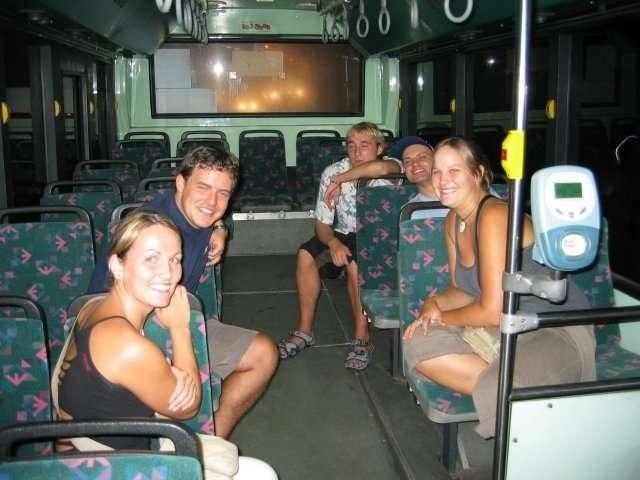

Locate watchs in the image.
[210,223,231,235]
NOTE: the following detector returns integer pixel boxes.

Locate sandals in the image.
[277,328,316,359]
[344,339,376,369]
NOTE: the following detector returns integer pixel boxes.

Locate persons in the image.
[403,137,597,441]
[282,121,406,371]
[390,135,502,218]
[86,145,278,440]
[48,211,278,480]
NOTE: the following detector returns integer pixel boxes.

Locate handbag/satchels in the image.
[462,325,500,364]
[159,427,239,479]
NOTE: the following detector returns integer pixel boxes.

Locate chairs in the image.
[228,128,297,216]
[295,126,640,471]
[1,126,228,480]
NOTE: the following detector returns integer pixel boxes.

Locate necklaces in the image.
[454,194,485,231]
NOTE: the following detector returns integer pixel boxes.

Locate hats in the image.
[387,135,435,160]
